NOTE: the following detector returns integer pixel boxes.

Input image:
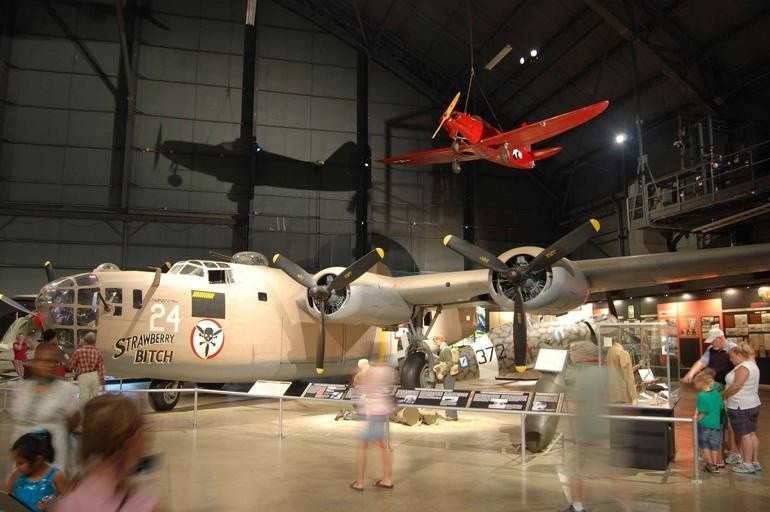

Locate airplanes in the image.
[372,91,612,171]
[384,292,643,385]
[0,213,770,415]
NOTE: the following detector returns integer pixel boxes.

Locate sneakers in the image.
[703,453,763,473]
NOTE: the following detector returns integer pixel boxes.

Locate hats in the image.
[704,329,723,343]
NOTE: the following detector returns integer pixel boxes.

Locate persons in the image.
[680,328,744,465]
[433,335,457,421]
[693,372,719,474]
[700,366,726,469]
[335,358,370,421]
[550,341,615,512]
[0,328,162,512]
[722,341,762,474]
[350,341,398,491]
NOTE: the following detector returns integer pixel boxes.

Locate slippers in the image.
[349,481,364,491]
[373,479,394,489]
